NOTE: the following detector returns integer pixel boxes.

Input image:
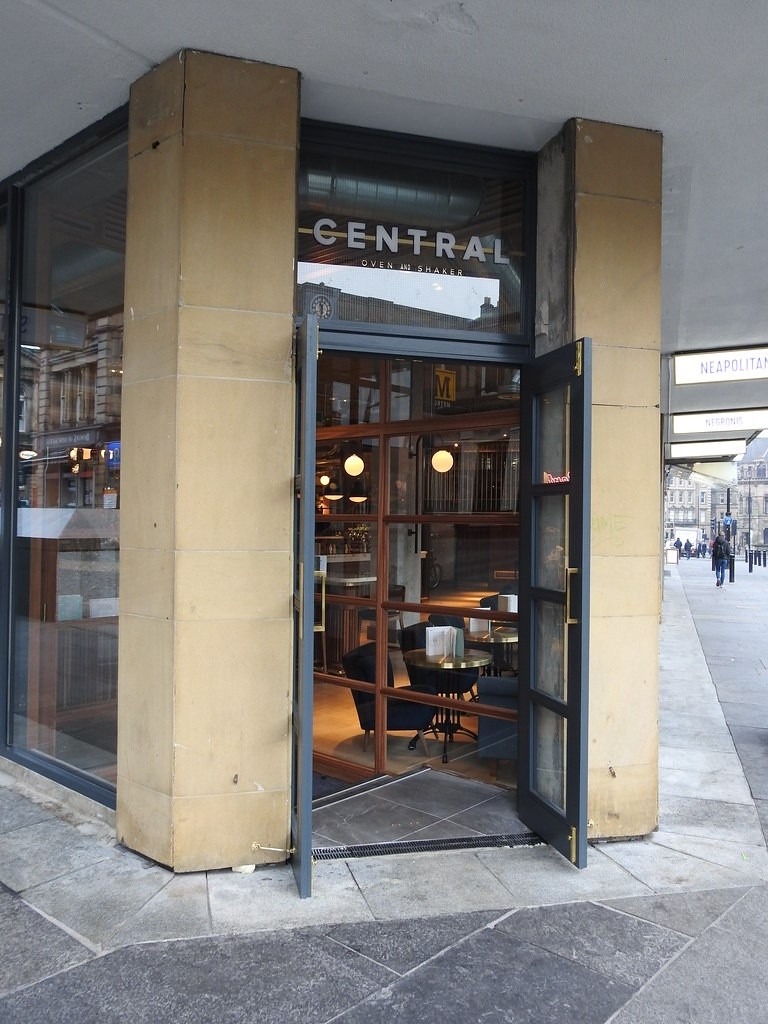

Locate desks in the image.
[402,649,494,764]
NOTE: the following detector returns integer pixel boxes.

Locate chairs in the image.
[426,591,519,678]
[342,640,439,757]
[357,584,406,646]
[399,620,434,690]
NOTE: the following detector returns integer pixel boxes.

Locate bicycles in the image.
[429,549,442,589]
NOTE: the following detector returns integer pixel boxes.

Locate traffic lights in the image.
[711,517,716,529]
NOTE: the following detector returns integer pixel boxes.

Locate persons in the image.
[674,538,682,560]
[713,532,730,587]
[702,542,706,556]
[697,542,704,558]
[737,543,741,555]
[684,539,692,559]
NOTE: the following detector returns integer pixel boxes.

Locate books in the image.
[425,626,464,657]
[470,607,492,633]
[498,594,518,613]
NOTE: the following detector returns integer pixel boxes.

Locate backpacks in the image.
[713,541,728,560]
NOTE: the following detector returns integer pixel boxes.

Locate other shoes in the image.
[720,583,723,588]
[716,578,720,586]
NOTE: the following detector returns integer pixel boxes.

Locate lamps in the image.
[348,477,368,503]
[322,470,344,501]
